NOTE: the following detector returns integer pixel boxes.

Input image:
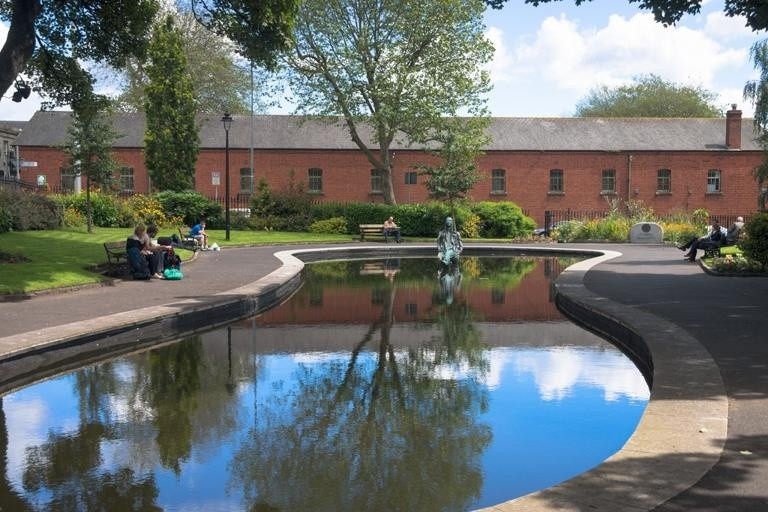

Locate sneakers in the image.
[677,245,696,262]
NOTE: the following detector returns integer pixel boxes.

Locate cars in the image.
[533,221,582,237]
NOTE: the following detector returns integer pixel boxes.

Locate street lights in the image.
[224,326,237,397]
[220,110,235,242]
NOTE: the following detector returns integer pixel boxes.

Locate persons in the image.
[683,221,722,263]
[436,267,463,305]
[676,218,720,257]
[724,215,745,243]
[383,215,400,243]
[435,215,463,266]
[141,226,167,280]
[126,224,151,280]
[189,220,209,252]
[382,259,400,284]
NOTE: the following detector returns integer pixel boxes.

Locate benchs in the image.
[697,224,740,259]
[359,224,401,243]
[176,225,201,250]
[102,236,163,265]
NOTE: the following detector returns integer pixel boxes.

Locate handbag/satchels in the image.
[163,267,183,280]
[164,251,180,270]
